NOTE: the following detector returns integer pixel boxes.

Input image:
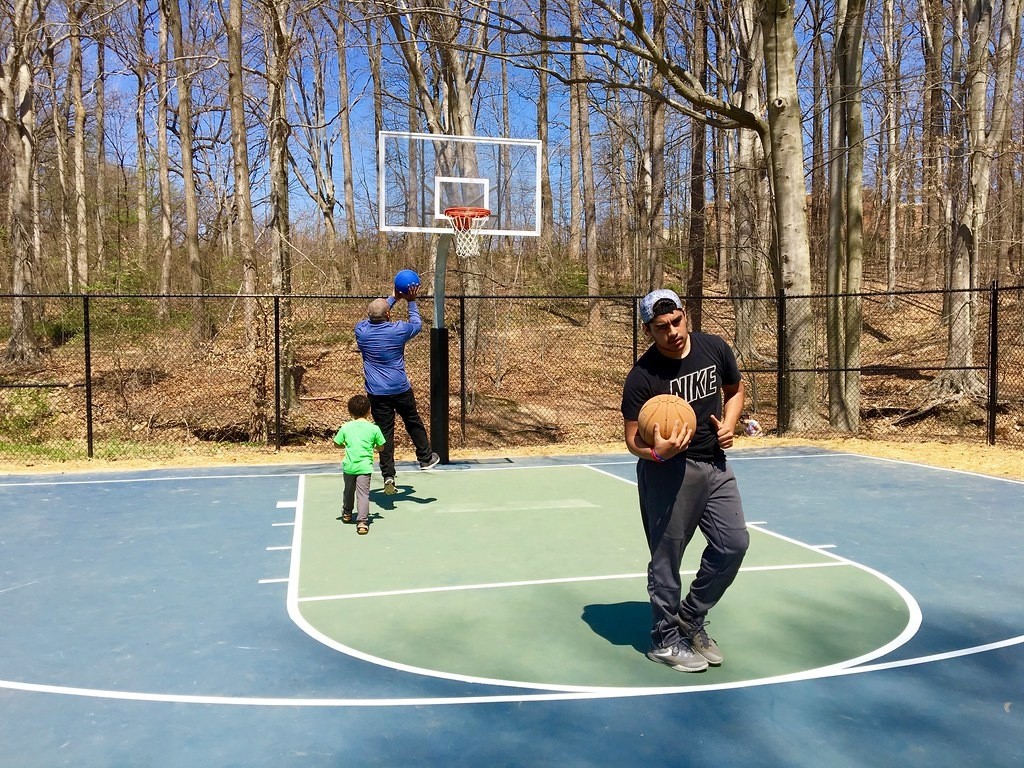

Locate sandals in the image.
[357,524,368,534]
[341,508,352,522]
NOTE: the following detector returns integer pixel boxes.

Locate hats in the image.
[640,289,683,324]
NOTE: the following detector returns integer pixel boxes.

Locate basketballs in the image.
[637,394,698,448]
[394,270,420,297]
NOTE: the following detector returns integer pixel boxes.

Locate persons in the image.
[333,394,387,536]
[358,282,440,498]
[620,289,750,675]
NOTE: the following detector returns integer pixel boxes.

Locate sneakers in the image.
[419,453,440,470]
[648,640,708,673]
[676,613,724,665]
[384,477,398,495]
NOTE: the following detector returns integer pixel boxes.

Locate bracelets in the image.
[651,447,666,464]
